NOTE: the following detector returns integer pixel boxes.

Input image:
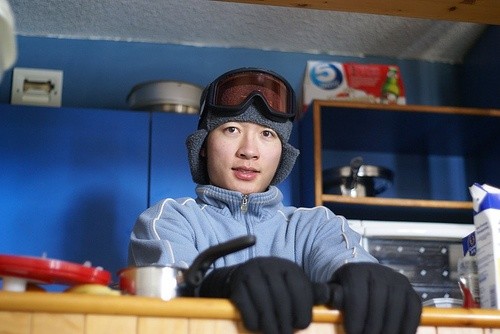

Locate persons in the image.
[124,68,422,334]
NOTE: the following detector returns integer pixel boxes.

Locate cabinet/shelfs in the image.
[0,102,303,307]
[301,102,500,221]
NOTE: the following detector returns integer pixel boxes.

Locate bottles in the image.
[380,65,399,105]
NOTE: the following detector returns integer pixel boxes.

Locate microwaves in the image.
[347,220,475,300]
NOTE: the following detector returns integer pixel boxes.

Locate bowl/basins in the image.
[116,265,187,302]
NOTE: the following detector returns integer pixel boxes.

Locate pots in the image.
[321,156,376,198]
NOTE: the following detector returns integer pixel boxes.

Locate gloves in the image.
[198,256,329,334]
[325,262,422,334]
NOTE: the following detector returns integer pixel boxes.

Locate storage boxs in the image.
[296,61,407,122]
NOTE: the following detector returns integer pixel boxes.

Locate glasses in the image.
[206,68,297,122]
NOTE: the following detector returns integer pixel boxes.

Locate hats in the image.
[185,67,300,186]
